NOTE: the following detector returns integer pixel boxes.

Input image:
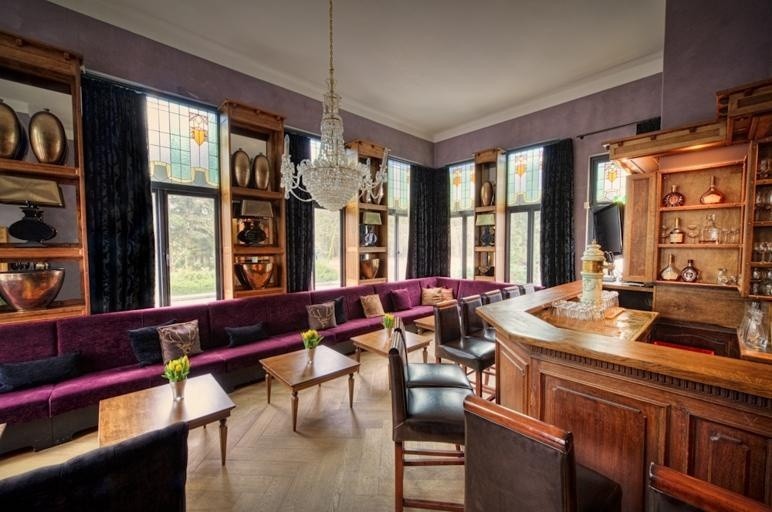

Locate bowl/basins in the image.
[0,268,64,311]
[235,262,274,290]
[361,258,380,278]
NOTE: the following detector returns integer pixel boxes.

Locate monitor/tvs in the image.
[592,201,624,255]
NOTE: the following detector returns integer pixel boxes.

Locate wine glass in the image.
[752,158,772,296]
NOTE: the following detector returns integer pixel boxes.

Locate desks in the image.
[349,327,433,374]
[96,370,236,465]
[413,314,435,337]
[257,344,362,431]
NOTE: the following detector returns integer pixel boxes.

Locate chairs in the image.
[647,461,772,511]
[388,332,474,512]
[463,393,623,510]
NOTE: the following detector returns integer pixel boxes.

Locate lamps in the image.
[280,0,390,213]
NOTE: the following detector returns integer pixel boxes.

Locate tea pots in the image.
[737,299,770,353]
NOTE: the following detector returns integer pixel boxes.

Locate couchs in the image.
[0,275,545,467]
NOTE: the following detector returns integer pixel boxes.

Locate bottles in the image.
[699,214,719,243]
[681,259,699,283]
[699,174,725,205]
[663,184,685,207]
[660,254,681,281]
[670,217,685,244]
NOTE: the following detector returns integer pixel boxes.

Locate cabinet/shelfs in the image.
[655,157,746,288]
[739,133,771,301]
[344,138,389,288]
[0,29,91,326]
[217,98,288,300]
[474,147,507,283]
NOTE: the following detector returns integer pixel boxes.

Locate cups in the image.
[552,289,620,322]
[717,225,739,243]
